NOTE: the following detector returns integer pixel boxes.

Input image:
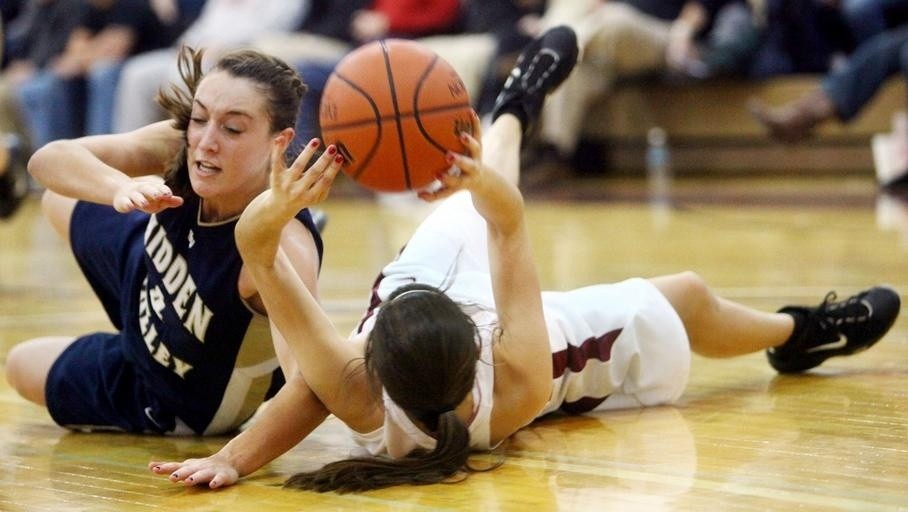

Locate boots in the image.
[746,89,833,144]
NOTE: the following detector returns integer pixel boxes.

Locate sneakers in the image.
[870,117,908,186]
[766,286,901,374]
[491,23,579,132]
[308,207,327,235]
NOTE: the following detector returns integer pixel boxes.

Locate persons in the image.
[5,42,332,490]
[1,0,908,192]
[233,26,900,494]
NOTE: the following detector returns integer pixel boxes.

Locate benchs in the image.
[496,47,908,144]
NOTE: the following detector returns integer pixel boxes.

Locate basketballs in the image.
[320,38,471,190]
